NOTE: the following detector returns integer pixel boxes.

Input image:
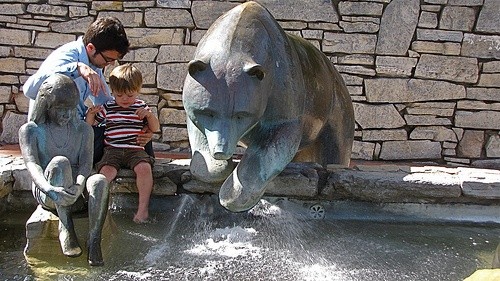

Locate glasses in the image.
[97,49,118,62]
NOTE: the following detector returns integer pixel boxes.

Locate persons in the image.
[23,14,155,168]
[85,62,160,225]
[18,73,109,266]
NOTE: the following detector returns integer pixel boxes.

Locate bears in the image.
[181,1,356,213]
